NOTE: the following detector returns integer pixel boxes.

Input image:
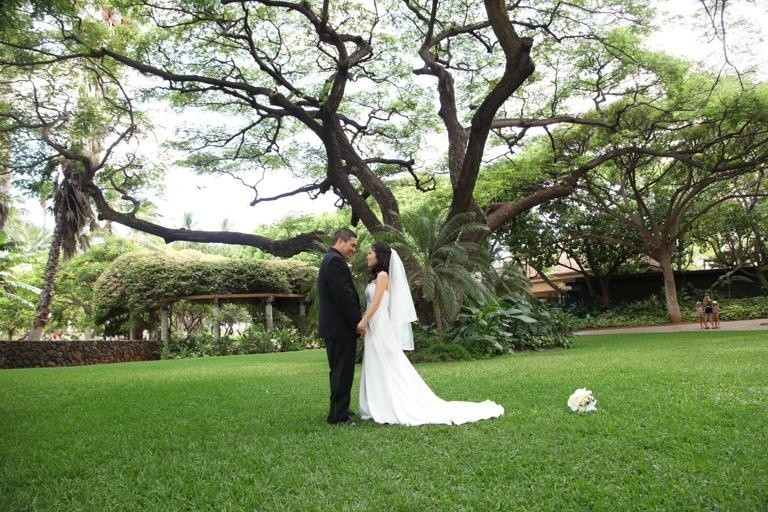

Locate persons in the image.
[315,228,364,424]
[696,302,707,330]
[711,298,720,330]
[358,240,407,423]
[702,292,713,328]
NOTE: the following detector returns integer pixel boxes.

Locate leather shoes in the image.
[347,408,354,416]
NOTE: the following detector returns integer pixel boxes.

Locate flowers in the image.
[567,386,597,414]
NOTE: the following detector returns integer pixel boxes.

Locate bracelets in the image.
[362,318,367,324]
